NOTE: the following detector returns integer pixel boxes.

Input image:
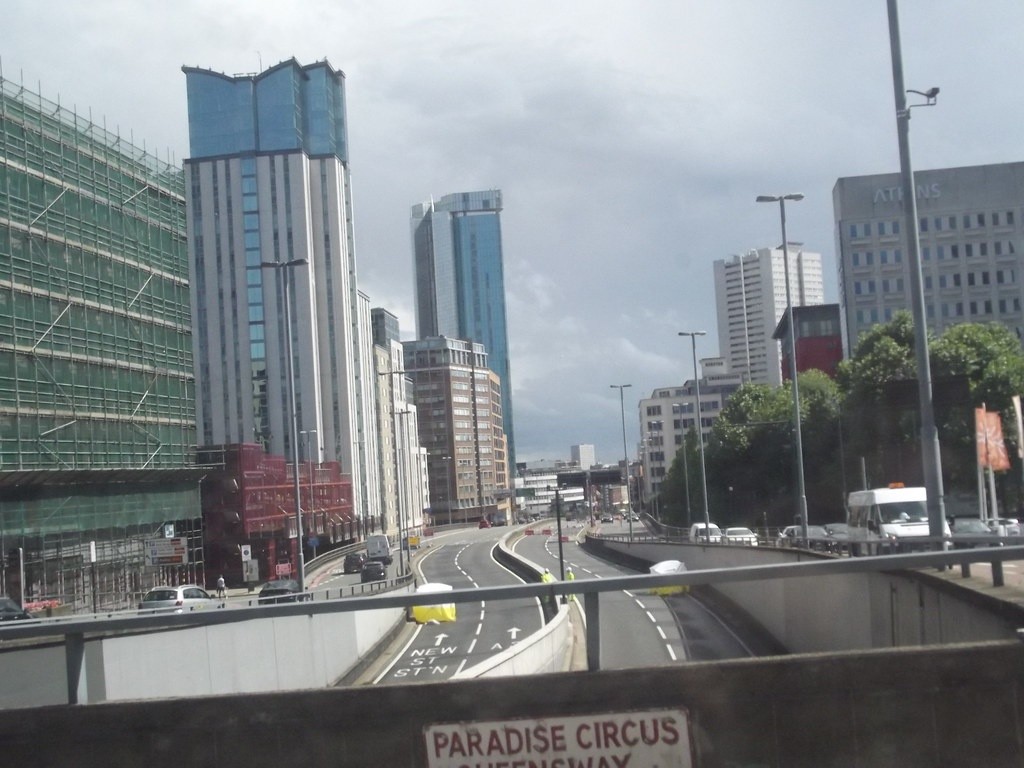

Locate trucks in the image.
[367,535,394,565]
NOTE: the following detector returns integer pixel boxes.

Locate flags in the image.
[976,396,1024,472]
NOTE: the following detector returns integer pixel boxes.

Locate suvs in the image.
[135,585,226,616]
[343,552,367,574]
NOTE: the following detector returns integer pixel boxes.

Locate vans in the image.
[688,522,723,544]
[848,483,955,570]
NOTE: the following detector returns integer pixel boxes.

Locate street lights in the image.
[609,383,635,540]
[679,330,714,543]
[546,483,568,604]
[757,192,816,552]
[257,256,308,598]
[378,369,408,576]
[390,410,413,565]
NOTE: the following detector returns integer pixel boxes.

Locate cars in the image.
[947,516,1003,550]
[776,521,883,559]
[722,527,758,547]
[479,520,491,529]
[601,513,615,523]
[986,516,1022,536]
[0,597,40,624]
[258,580,309,606]
[360,562,388,583]
[623,510,640,522]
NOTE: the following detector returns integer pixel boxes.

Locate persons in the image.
[564,567,575,603]
[540,568,553,603]
[216,573,226,598]
